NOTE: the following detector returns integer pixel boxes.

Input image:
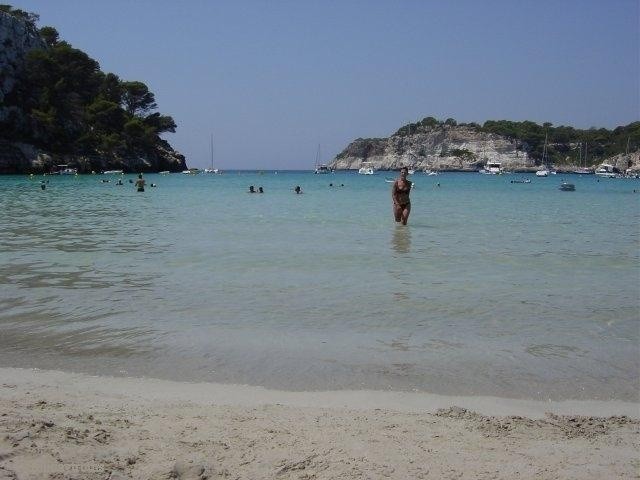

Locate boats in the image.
[485,162,502,174]
[358,162,374,176]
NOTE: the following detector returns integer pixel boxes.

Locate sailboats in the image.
[536,133,549,177]
[573,142,593,174]
[205,132,221,173]
[315,144,332,174]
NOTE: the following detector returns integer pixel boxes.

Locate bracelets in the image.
[396,203,400,206]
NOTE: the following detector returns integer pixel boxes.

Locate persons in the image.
[134,173,147,192]
[294,186,301,194]
[258,186,264,194]
[101,176,157,189]
[391,166,412,227]
[247,186,256,194]
